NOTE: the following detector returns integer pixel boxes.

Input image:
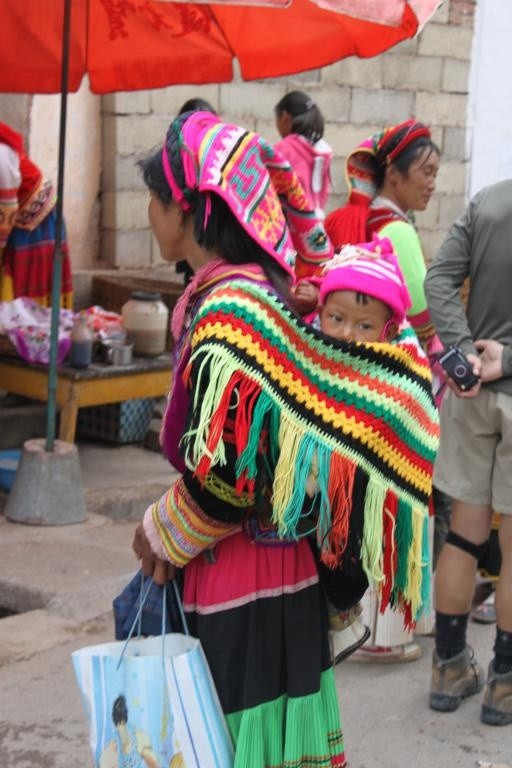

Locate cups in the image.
[100,338,137,366]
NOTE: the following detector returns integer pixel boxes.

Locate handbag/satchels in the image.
[71,635,236,768]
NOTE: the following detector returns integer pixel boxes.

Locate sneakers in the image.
[327,622,371,665]
[429,645,484,712]
[348,645,421,666]
[481,659,511,725]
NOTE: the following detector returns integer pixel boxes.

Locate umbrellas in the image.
[0,0,438,450]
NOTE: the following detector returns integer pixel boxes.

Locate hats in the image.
[320,236,412,325]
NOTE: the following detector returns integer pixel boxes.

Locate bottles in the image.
[69,309,96,366]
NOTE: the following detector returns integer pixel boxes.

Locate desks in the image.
[1,351,176,445]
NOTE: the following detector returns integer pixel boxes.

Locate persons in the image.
[1,123,71,311]
[295,240,409,663]
[425,179,511,724]
[272,88,329,214]
[329,119,444,659]
[131,110,344,766]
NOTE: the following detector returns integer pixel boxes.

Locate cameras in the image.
[438,344,481,392]
